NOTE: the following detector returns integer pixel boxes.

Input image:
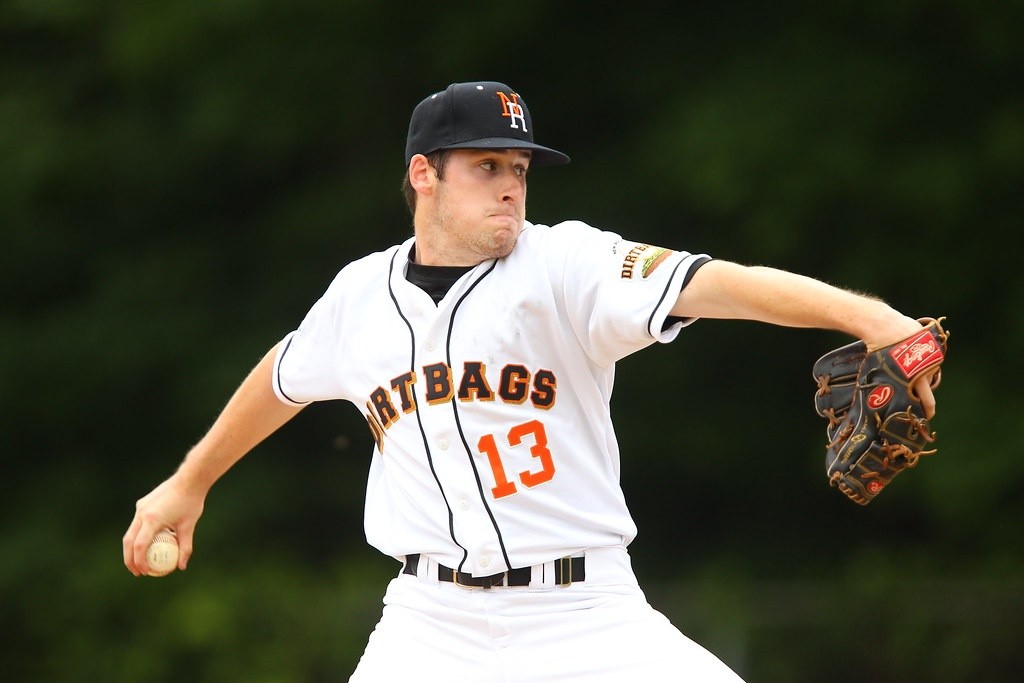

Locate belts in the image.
[404,554,584,586]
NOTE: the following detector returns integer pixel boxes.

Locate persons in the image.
[122,81,938,683]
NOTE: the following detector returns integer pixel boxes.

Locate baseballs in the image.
[146,531,181,578]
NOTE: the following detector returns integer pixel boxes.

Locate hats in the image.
[405,81,571,164]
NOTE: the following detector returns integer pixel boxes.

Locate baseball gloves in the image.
[812,314,950,507]
